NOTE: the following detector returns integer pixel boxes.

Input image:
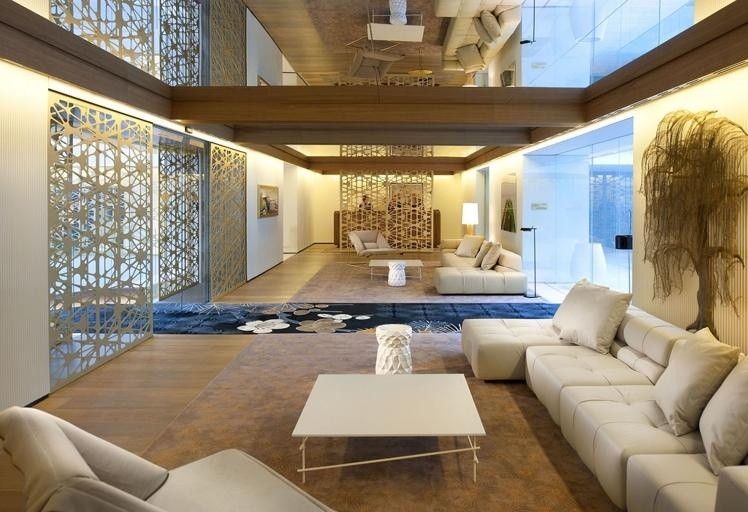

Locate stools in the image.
[374,322,414,374]
[386,0,408,25]
[387,262,407,287]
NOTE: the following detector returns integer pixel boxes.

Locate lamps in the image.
[614,234,632,293]
[461,202,480,235]
[520,225,541,298]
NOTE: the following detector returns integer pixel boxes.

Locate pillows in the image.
[556,284,635,356]
[651,325,741,439]
[455,10,504,74]
[454,234,486,258]
[551,277,609,331]
[698,353,748,476]
[480,239,503,271]
[469,241,494,268]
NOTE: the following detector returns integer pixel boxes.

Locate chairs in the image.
[345,229,404,271]
[0,403,340,512]
[345,38,405,82]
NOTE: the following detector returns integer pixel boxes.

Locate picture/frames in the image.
[256,183,280,220]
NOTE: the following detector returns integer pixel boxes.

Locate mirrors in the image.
[11,0,737,90]
[151,120,637,304]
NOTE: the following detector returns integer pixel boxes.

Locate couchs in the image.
[431,238,528,296]
[461,304,748,511]
[433,1,523,72]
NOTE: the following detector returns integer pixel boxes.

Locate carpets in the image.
[301,1,453,76]
[140,333,626,512]
[286,261,551,304]
[55,299,562,335]
